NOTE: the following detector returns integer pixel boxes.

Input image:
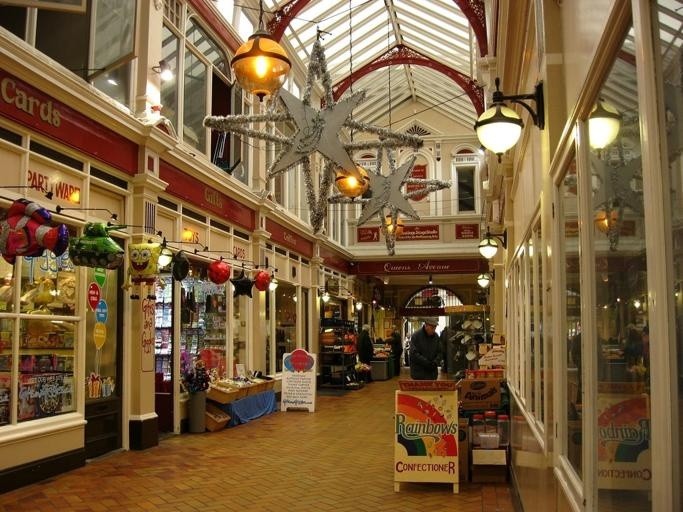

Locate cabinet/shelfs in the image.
[318,318,355,390]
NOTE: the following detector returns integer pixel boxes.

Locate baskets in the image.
[206,371,274,404]
[203,404,231,432]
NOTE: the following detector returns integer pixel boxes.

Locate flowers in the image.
[179,351,210,394]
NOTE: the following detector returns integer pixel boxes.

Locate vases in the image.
[188,390,206,434]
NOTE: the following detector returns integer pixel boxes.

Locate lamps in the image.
[476,268,495,289]
[575,96,623,150]
[318,288,330,302]
[596,209,619,237]
[353,299,362,310]
[478,225,507,259]
[379,17,404,236]
[152,60,173,85]
[231,0,292,103]
[269,278,279,291]
[473,77,544,162]
[334,0,370,202]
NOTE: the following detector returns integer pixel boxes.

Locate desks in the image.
[394,389,470,494]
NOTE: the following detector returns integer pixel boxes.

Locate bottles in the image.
[484,410,497,433]
[496,415,509,446]
[472,414,485,446]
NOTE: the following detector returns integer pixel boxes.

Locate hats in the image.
[424,317,439,327]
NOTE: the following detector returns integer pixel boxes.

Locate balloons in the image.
[208,261,229,284]
[1,197,68,267]
[120,242,166,300]
[230,269,254,299]
[68,222,127,270]
[254,271,269,292]
[172,252,189,281]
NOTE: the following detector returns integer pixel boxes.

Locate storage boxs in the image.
[461,369,503,411]
[206,379,282,432]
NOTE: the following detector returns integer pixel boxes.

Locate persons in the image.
[357,325,374,383]
[408,319,443,380]
[387,332,403,376]
[341,328,356,369]
[439,327,447,373]
[624,329,643,394]
[642,326,649,370]
